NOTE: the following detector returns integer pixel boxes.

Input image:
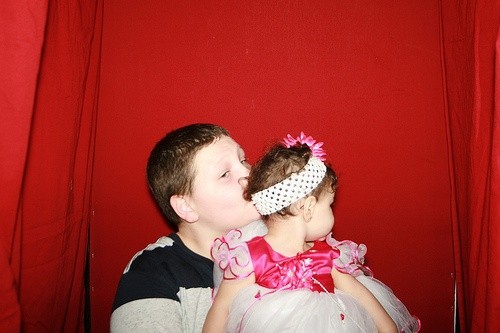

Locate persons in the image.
[201,142,398,333]
[111,120,261,333]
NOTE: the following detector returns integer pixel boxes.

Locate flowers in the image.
[281,132,327,162]
[280,258,312,289]
